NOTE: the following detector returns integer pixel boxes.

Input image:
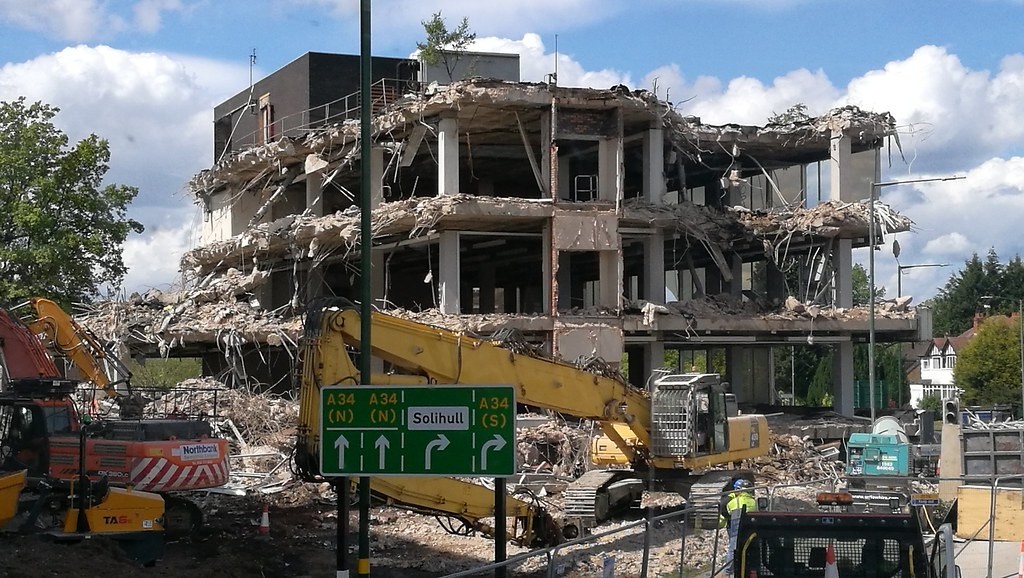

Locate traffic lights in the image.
[944,398,958,424]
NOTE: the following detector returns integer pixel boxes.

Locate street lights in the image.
[868,172,967,425]
[897,262,954,415]
[980,294,1023,421]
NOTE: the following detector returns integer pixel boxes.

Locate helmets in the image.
[740,480,753,493]
[734,479,744,488]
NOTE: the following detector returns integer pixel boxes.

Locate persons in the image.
[856,538,896,577]
[718,479,758,578]
[16,438,41,470]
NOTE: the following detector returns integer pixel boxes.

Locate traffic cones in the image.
[824,544,840,578]
[258,502,271,534]
[935,458,941,474]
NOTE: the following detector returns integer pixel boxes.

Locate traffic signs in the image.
[320,382,517,480]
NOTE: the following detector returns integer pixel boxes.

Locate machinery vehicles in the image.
[287,293,769,549]
[0,295,231,549]
[834,414,916,493]
